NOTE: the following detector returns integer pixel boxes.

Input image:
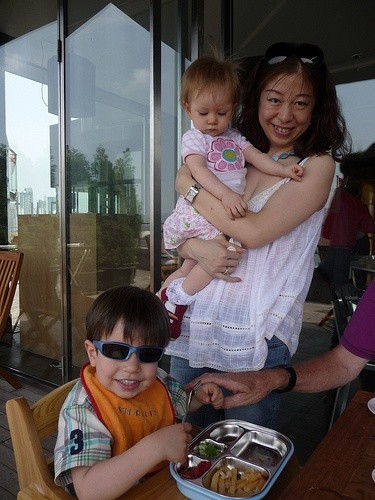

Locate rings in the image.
[222,267,228,275]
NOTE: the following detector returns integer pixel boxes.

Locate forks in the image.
[181,380,203,423]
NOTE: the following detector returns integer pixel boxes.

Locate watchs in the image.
[183,183,204,205]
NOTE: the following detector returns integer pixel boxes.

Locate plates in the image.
[367,398,375,416]
[170,418,295,500]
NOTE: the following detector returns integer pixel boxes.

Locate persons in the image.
[157,55,305,338]
[321,175,375,302]
[150,42,353,426]
[52,285,224,500]
[182,276,375,410]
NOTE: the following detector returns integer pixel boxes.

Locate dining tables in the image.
[114,390,375,500]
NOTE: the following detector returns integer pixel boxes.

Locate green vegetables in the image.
[194,442,222,459]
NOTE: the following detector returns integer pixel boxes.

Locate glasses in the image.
[93,340,167,363]
[264,41,324,67]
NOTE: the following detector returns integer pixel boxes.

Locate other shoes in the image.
[161,286,187,340]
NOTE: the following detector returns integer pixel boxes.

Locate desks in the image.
[349,254,375,292]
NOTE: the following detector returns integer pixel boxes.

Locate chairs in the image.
[140,232,181,291]
[6,374,80,500]
[325,286,375,434]
[0,250,25,343]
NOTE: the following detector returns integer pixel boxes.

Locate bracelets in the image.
[271,364,296,394]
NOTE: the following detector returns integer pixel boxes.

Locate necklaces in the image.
[270,150,297,162]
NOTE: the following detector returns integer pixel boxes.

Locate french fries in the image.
[211,465,266,497]
